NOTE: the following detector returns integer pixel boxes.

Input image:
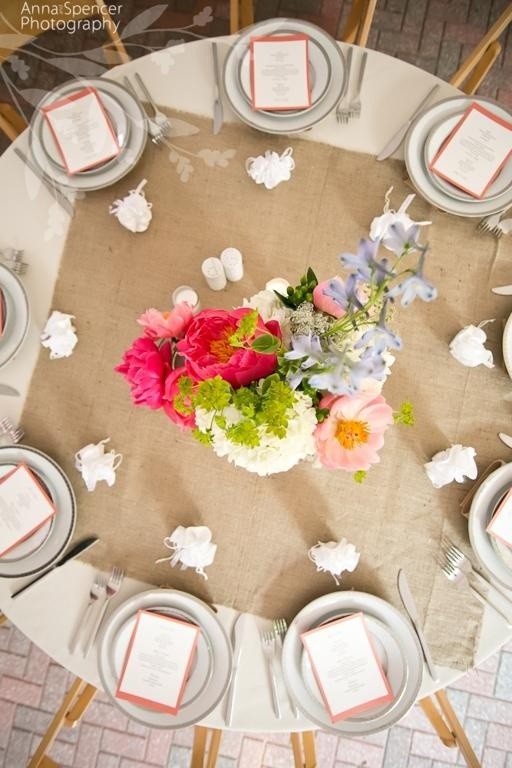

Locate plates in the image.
[1,16,512,736]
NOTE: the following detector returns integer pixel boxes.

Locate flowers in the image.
[113,227,440,479]
